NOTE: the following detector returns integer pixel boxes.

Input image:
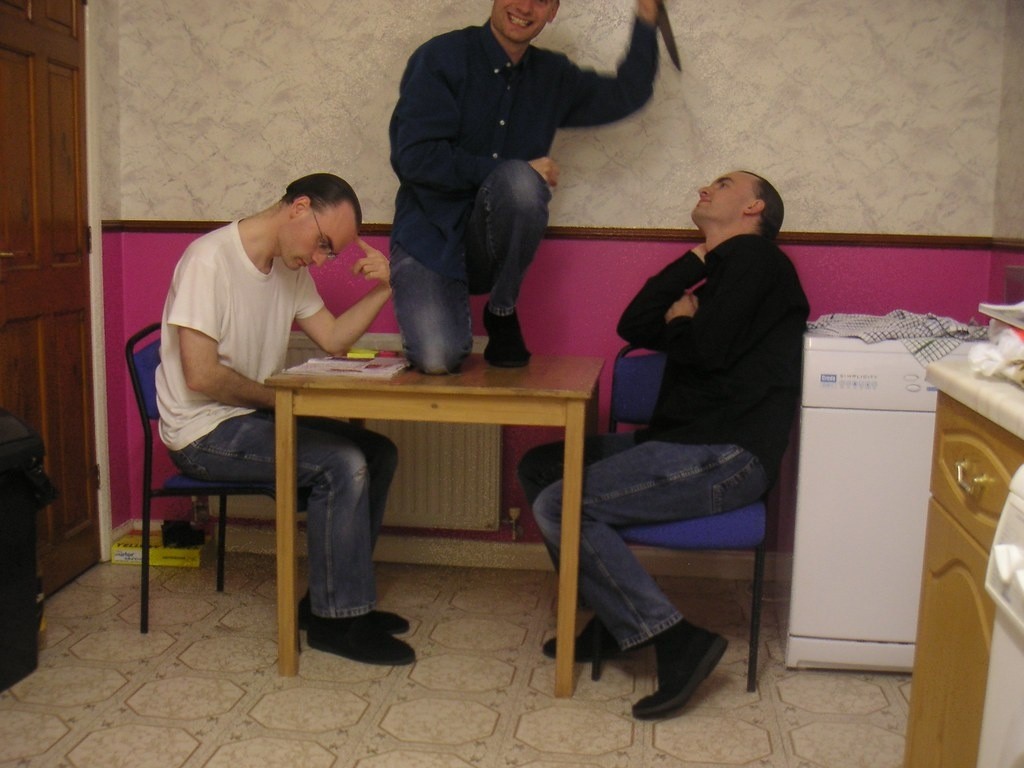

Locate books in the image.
[281,356,411,377]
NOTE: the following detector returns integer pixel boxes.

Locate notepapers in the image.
[346,348,380,360]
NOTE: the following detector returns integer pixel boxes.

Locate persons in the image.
[154,173,419,666]
[520,169,810,721]
[389,0,662,377]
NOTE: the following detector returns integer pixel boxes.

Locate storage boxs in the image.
[111,532,215,568]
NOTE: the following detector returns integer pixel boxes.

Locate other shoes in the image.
[543,614,620,662]
[482,302,528,366]
[298,589,410,634]
[307,614,416,666]
[632,628,729,719]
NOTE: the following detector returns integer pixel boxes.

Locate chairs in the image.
[590,343,772,694]
[124,322,312,656]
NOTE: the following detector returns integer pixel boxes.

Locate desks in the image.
[901,360,1024,768]
[263,350,607,698]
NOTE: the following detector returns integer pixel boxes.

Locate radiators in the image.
[207,329,503,533]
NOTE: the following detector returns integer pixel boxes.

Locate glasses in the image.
[310,205,335,260]
